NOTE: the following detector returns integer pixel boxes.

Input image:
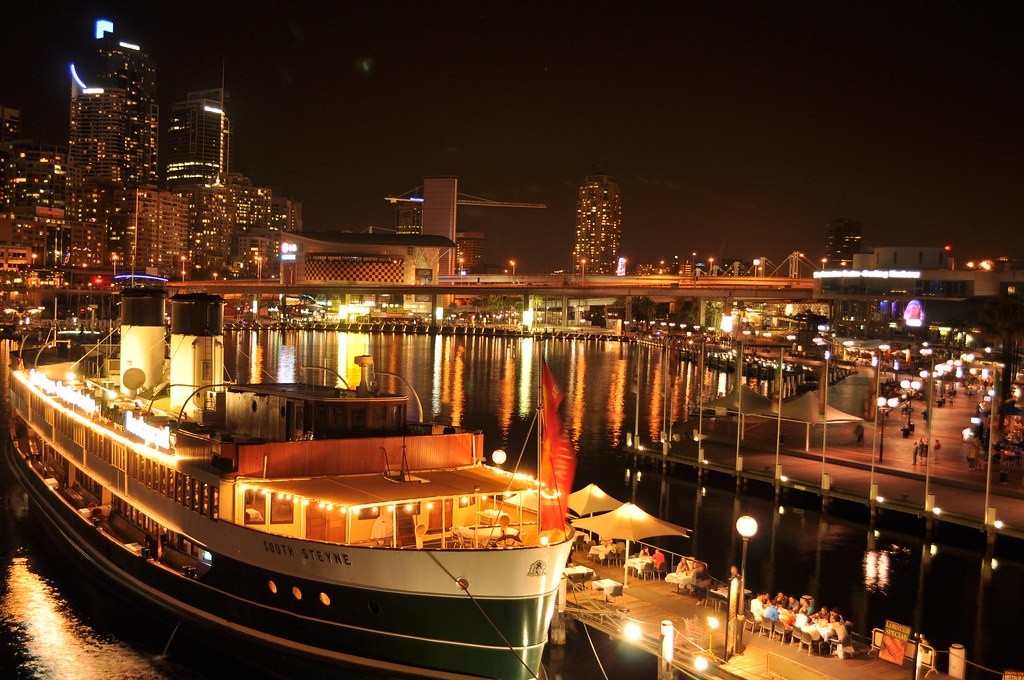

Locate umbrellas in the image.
[565,482,624,541]
[759,390,864,451]
[570,501,694,588]
[697,383,778,440]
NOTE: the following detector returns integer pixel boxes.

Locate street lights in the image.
[945,246,954,270]
[752,259,828,278]
[254,256,263,283]
[113,255,117,291]
[660,260,665,284]
[460,257,464,287]
[709,258,713,277]
[510,261,516,276]
[877,354,995,463]
[624,319,933,374]
[31,254,38,289]
[582,259,585,287]
[181,255,186,282]
[443,312,587,331]
[736,516,758,653]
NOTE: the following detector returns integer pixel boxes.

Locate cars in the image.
[298,303,324,318]
[290,307,314,319]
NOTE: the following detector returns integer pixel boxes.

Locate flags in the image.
[538,353,578,532]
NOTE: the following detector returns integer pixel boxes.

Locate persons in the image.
[912,437,985,471]
[637,545,851,658]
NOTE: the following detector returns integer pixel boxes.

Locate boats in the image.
[5,289,576,680]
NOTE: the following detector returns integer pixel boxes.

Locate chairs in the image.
[572,534,854,660]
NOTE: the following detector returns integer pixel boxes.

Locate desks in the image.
[592,579,623,603]
[422,531,453,542]
[564,565,593,591]
[574,531,590,543]
[664,573,693,593]
[587,545,616,565]
[479,511,501,525]
[624,556,653,578]
[709,587,752,612]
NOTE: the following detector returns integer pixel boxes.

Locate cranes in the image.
[383,185,546,209]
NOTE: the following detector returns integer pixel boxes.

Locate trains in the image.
[223,293,324,316]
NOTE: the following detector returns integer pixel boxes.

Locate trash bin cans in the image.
[903,425,909,438]
[1000,470,1008,484]
